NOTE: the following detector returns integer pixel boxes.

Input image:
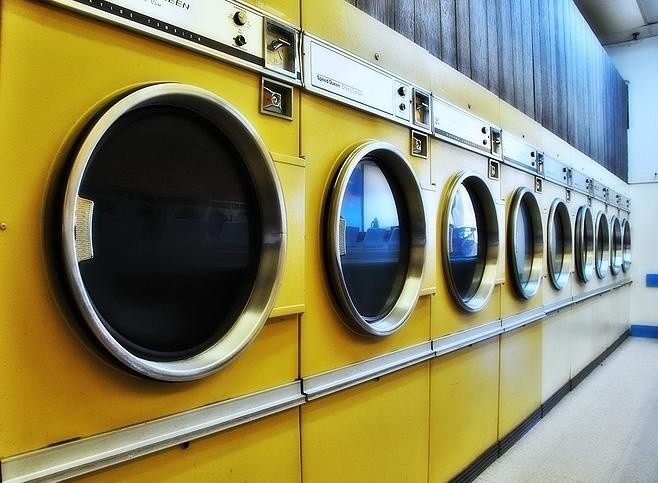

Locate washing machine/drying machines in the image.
[0,0,307,482]
[300,1,431,482]
[436,1,635,481]
